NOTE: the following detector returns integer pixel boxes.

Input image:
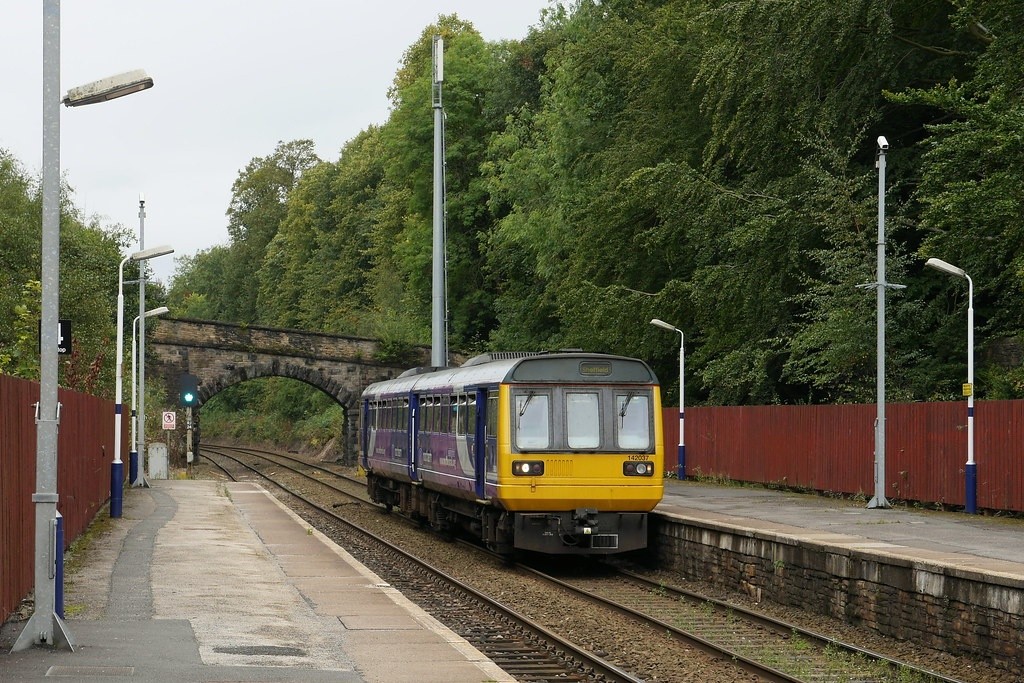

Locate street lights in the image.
[924,256,978,514]
[649,318,688,481]
[56,68,159,623]
[127,306,169,484]
[109,244,175,520]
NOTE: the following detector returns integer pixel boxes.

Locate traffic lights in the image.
[180,374,199,407]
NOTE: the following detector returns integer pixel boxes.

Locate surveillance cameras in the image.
[139,193,144,204]
[877,136,889,149]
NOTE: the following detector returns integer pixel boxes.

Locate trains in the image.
[356,350,665,566]
